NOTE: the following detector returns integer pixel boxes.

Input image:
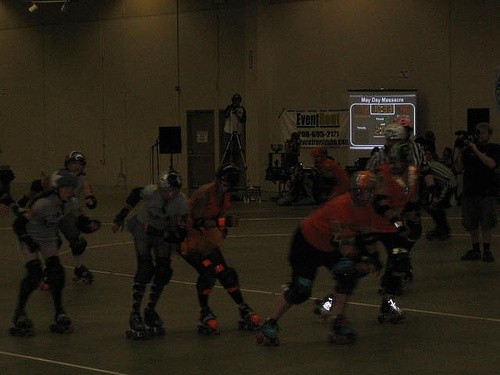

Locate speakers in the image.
[158,126,182,154]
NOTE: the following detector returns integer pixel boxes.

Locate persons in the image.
[112,172,190,340]
[0,151,101,335]
[255,114,500,347]
[179,163,264,338]
[222,94,246,160]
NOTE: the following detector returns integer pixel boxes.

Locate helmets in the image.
[50,169,79,191]
[163,172,182,191]
[216,164,240,183]
[393,115,413,129]
[312,147,321,158]
[350,171,376,191]
[64,151,86,170]
[384,124,407,144]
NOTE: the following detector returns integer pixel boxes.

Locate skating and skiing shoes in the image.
[238,304,261,330]
[39,269,50,290]
[72,265,94,284]
[198,307,221,335]
[377,297,405,323]
[50,313,72,334]
[314,294,334,317]
[143,311,165,336]
[8,309,34,336]
[326,314,358,344]
[256,317,280,346]
[125,313,151,340]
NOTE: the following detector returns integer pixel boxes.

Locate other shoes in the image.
[425,226,437,239]
[460,250,481,260]
[436,225,450,241]
[482,250,495,261]
